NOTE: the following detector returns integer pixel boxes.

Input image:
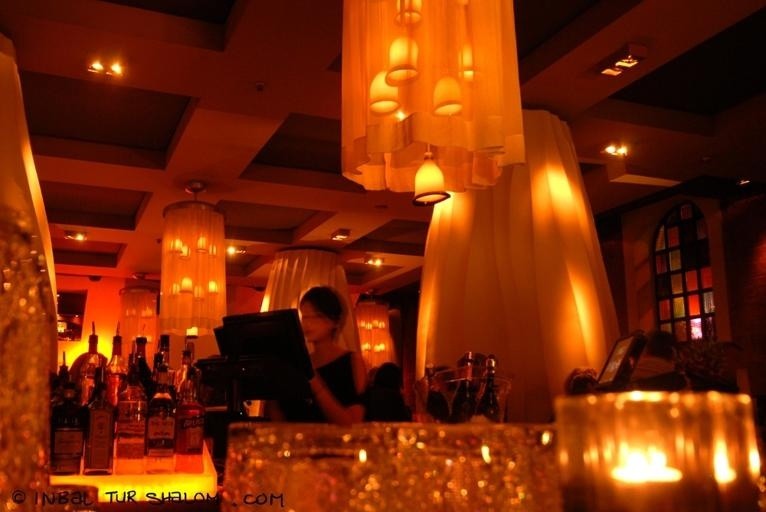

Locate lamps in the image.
[350,297,395,371]
[555,389,766,512]
[597,45,639,77]
[260,244,364,395]
[158,183,227,339]
[417,108,618,422]
[339,0,525,205]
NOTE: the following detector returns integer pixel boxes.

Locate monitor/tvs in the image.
[214,309,314,399]
[594,333,647,388]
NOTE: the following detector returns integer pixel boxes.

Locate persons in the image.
[624,331,691,391]
[266,287,412,425]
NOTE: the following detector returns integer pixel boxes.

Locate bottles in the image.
[53,334,205,476]
[424,351,500,421]
